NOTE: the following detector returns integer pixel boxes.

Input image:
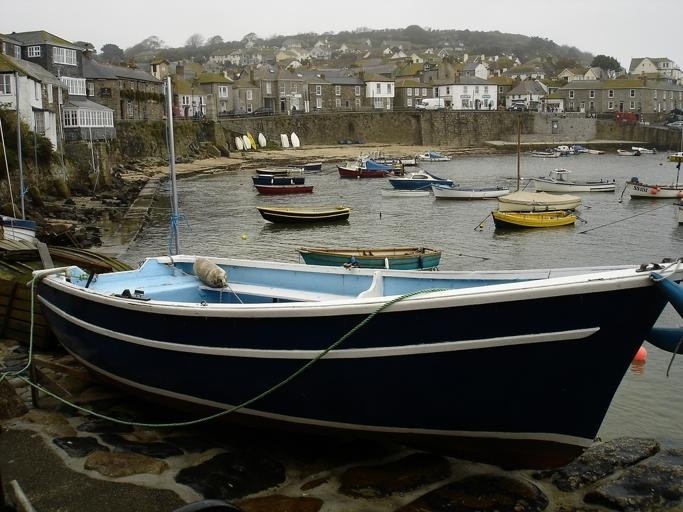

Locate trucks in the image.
[414,98,445,112]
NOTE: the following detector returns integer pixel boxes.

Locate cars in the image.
[667,121,683,131]
[587,111,596,118]
[219,107,274,118]
[508,103,538,112]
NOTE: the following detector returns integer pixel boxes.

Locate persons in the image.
[489,102,491,110]
[551,106,557,112]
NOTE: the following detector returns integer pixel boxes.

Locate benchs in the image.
[199,272,384,303]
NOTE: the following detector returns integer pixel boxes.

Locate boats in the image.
[34,77,683,472]
[254,200,351,225]
[251,159,324,194]
[232,130,300,150]
[0,213,138,353]
[293,242,442,271]
